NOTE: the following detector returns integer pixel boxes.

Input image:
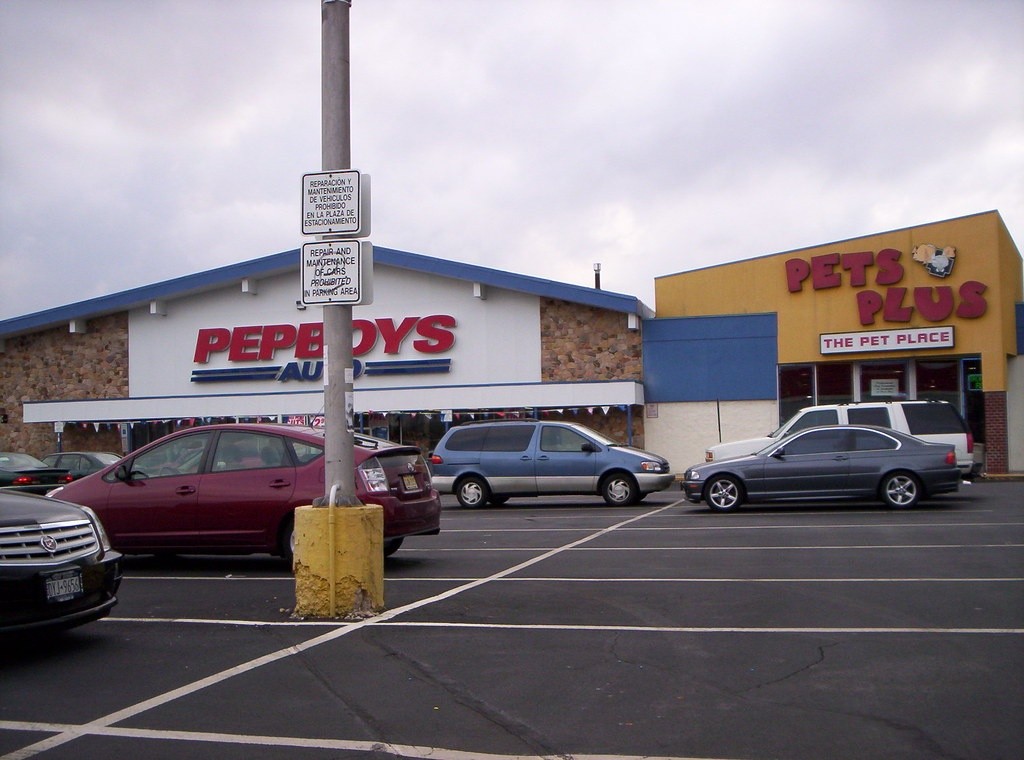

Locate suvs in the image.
[705,395,976,479]
[42,422,444,565]
[428,416,676,512]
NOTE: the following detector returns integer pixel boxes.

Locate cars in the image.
[39,450,124,480]
[0,485,125,639]
[1,450,73,497]
[678,423,962,513]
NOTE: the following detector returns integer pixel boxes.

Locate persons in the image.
[234,440,267,466]
